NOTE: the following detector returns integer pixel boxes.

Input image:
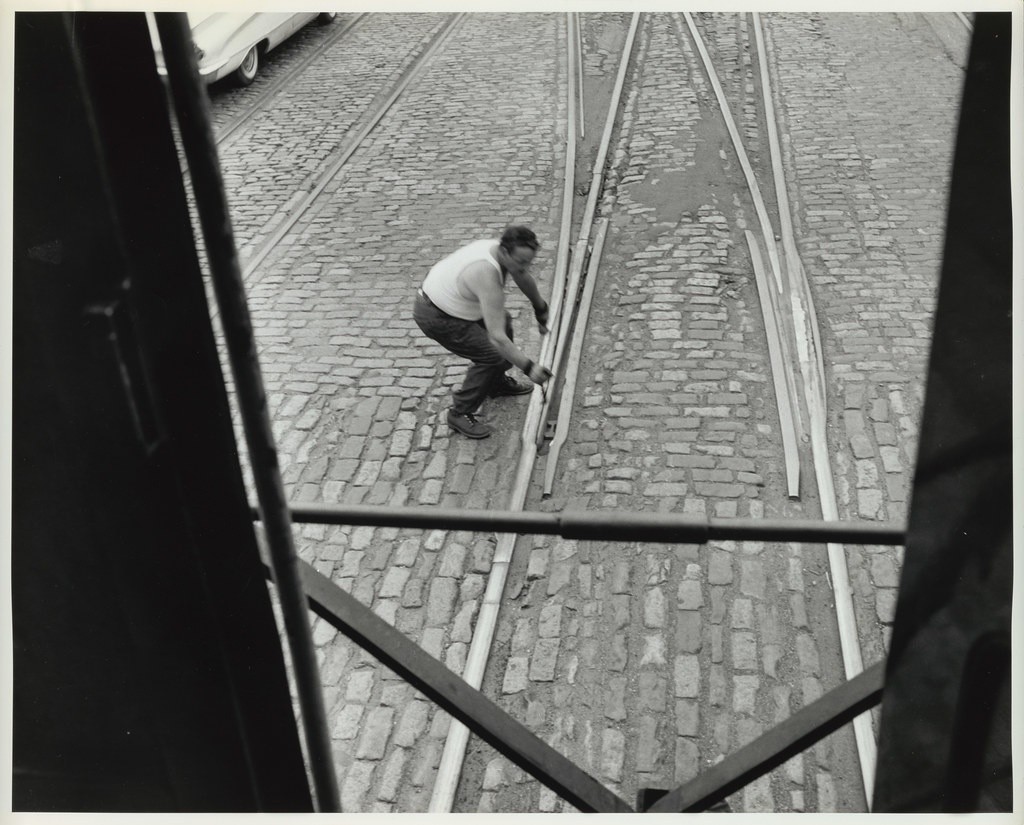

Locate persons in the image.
[415,227,554,439]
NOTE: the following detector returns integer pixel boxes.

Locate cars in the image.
[139,11,337,89]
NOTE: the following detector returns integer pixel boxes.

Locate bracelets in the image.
[524,359,534,376]
[533,300,547,314]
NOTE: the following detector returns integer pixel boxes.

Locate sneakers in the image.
[447,408,491,438]
[490,375,534,396]
